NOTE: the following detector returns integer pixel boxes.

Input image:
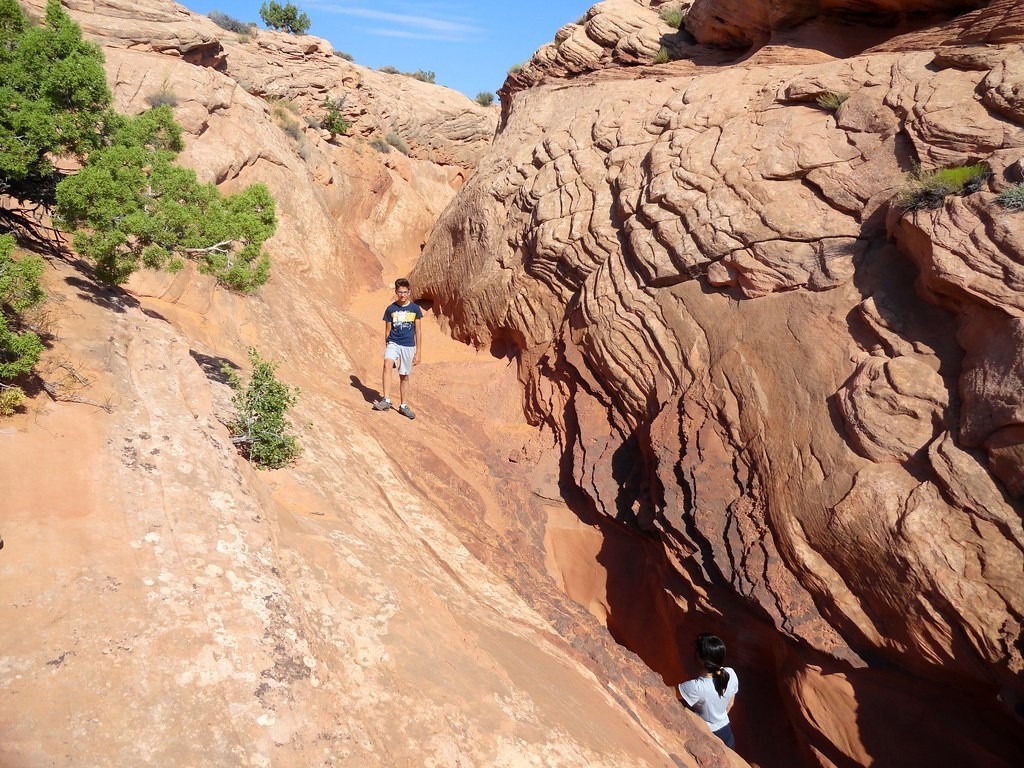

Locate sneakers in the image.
[399,405,415,419]
[374,399,391,411]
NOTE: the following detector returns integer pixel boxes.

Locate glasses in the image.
[692,640,697,650]
[395,290,409,295]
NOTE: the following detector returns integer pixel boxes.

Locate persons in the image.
[672,633,739,757]
[373,278,423,419]
